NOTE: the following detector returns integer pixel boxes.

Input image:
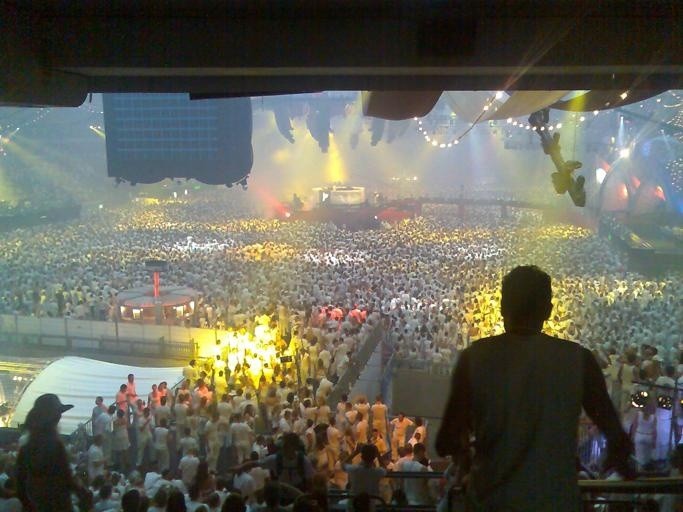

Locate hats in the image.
[35,394,73,415]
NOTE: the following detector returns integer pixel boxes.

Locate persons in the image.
[1,125,683,512]
